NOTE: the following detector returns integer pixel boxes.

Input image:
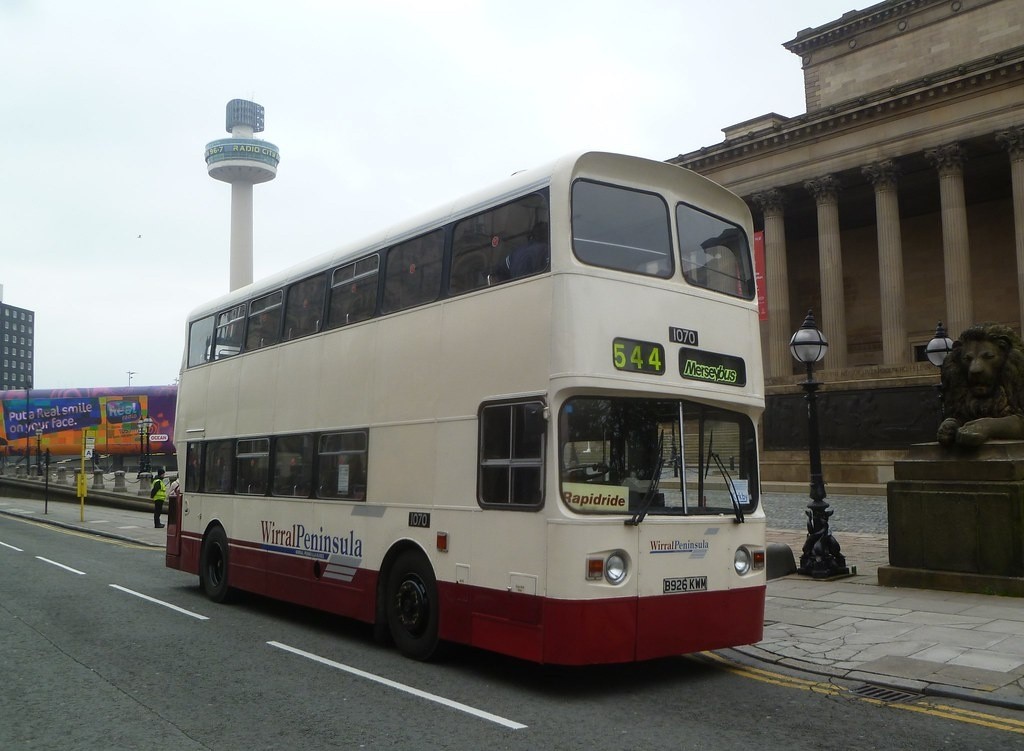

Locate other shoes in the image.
[155,524,165,528]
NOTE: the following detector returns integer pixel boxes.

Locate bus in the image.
[164,149,767,665]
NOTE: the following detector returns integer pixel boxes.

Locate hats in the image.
[157,468,165,475]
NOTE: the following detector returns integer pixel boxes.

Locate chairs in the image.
[218,255,513,360]
[248,482,364,497]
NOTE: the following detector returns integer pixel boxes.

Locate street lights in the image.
[137,415,146,479]
[924,320,955,420]
[143,414,154,472]
[788,308,850,580]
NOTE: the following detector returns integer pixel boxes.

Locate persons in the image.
[151,469,166,528]
[168,472,179,495]
